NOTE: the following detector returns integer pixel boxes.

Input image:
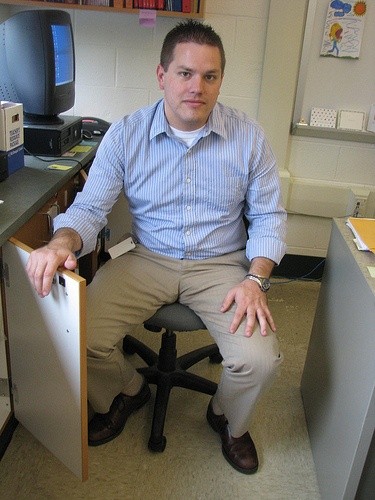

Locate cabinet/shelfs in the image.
[0,0,207,20]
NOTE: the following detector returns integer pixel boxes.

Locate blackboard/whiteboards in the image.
[289,0,375,143]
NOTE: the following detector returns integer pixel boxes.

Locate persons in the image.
[27,17,286,475]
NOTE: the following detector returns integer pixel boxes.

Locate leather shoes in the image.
[207,397,258,475]
[88,381,151,446]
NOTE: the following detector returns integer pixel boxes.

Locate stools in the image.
[122,302,223,453]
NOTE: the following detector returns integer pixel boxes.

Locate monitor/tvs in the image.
[1,8,76,124]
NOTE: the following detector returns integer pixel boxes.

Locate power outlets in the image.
[344,188,371,218]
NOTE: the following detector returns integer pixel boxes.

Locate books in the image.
[0,100,24,151]
[35,0,199,14]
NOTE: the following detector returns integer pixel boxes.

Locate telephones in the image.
[82,117,110,137]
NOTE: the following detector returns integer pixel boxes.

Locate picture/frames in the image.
[337,109,366,132]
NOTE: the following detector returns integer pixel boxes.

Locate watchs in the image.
[245,274,271,292]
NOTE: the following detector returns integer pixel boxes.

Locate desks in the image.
[300,217,375,500]
[0,142,99,482]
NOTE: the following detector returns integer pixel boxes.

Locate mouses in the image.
[81,129,93,139]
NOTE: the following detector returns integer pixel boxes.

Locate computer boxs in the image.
[22,115,84,157]
